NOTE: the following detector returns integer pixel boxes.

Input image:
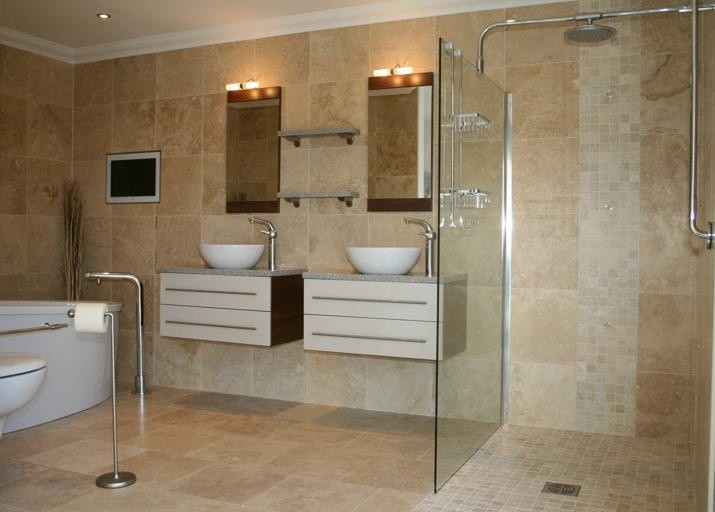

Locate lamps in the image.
[373,68,393,77]
[394,66,413,75]
[225,82,243,91]
[245,81,259,89]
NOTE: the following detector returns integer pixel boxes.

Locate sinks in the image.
[345,245,422,274]
[197,243,264,268]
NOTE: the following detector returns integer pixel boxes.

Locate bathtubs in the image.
[0,299,124,436]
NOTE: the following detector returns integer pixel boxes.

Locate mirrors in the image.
[368,85,432,199]
[226,99,280,201]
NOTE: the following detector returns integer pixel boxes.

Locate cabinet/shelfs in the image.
[303,280,467,361]
[159,272,303,347]
[277,127,361,207]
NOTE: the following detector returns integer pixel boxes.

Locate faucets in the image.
[249,215,277,272]
[84,272,147,395]
[404,216,435,275]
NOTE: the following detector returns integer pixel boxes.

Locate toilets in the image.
[0,355,47,425]
